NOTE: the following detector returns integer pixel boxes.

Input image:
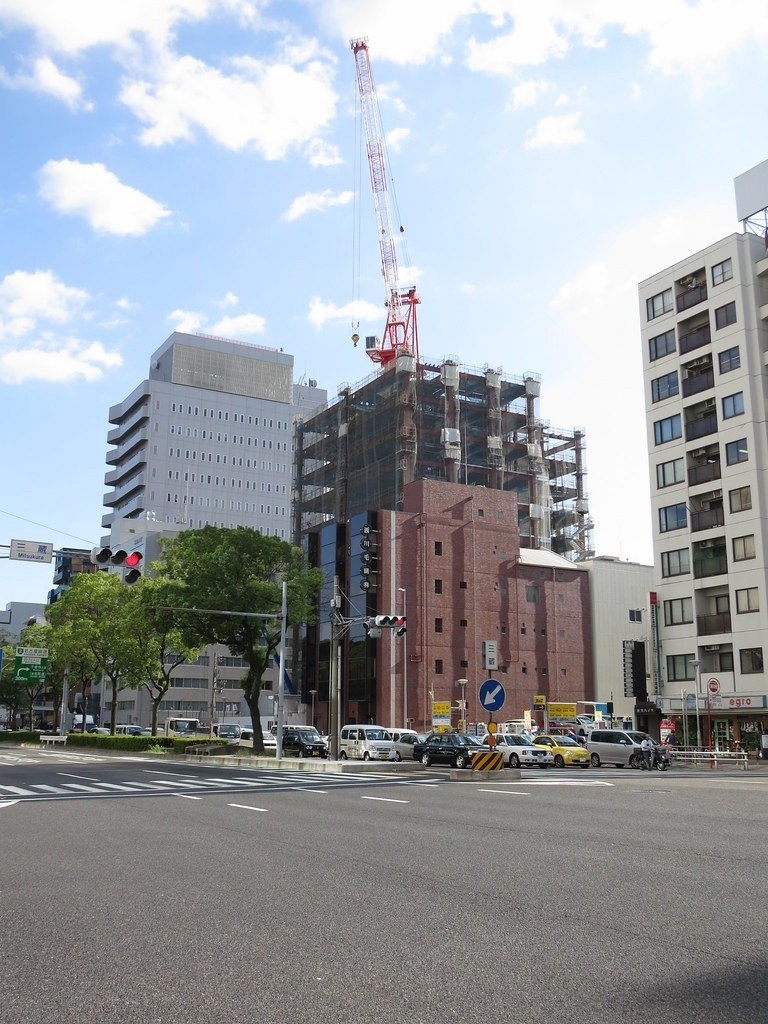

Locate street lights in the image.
[458,679,468,734]
[309,690,318,727]
[688,660,702,755]
[397,587,408,729]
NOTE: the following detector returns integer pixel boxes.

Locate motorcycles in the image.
[634,747,666,771]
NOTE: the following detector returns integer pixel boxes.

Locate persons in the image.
[641,734,652,770]
[665,730,677,746]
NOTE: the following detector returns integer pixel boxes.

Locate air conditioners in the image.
[705,397,716,408]
[692,447,706,457]
[699,539,712,549]
[687,355,709,368]
[705,645,719,651]
[680,275,693,285]
[712,489,722,498]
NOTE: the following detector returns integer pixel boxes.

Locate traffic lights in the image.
[90,547,144,568]
[375,615,407,628]
[393,626,407,637]
[122,568,142,586]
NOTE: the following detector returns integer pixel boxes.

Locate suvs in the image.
[282,730,327,758]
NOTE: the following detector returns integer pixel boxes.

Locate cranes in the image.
[349,35,428,383]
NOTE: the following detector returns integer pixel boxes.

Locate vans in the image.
[270,724,320,740]
[378,728,418,743]
[586,729,658,769]
[341,724,395,761]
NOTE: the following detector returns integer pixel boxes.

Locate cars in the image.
[395,733,446,762]
[413,733,499,769]
[481,733,555,769]
[567,716,597,736]
[532,735,592,768]
[16,714,277,749]
[504,719,586,744]
